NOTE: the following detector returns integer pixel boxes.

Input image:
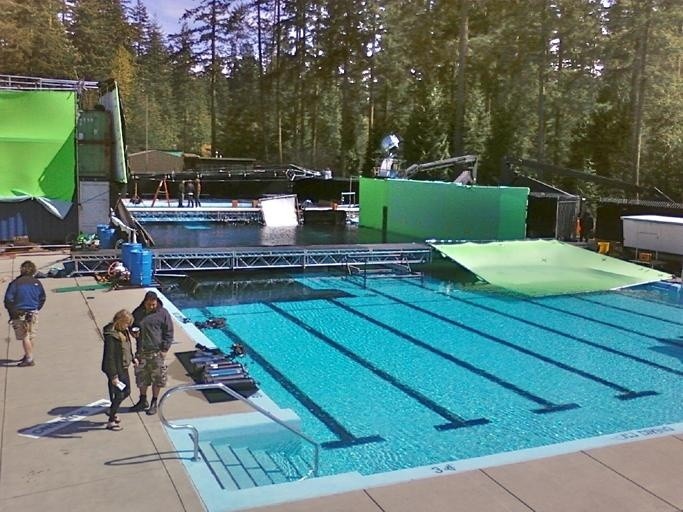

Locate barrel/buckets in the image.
[128,249,154,287]
[97,223,110,235]
[120,243,142,266]
[99,229,115,249]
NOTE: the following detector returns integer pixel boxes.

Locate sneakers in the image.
[128,400,150,411]
[17,354,35,367]
[146,400,157,416]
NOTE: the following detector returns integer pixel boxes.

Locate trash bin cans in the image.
[121,242,152,287]
[97,224,115,249]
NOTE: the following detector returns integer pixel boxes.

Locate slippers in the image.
[104,407,124,432]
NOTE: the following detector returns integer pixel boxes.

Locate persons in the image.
[177,180,185,207]
[127,290,173,416]
[101,310,138,432]
[453,169,477,186]
[322,167,333,180]
[390,158,407,179]
[3,260,44,366]
[578,211,593,243]
[186,178,194,207]
[194,177,201,207]
